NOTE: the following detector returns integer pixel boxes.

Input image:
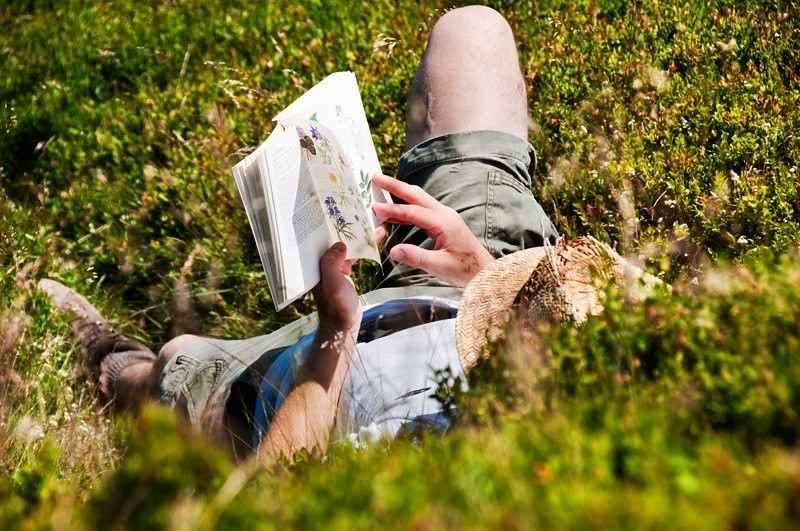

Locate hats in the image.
[454,219,675,419]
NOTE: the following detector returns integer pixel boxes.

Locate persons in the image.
[39,5,676,463]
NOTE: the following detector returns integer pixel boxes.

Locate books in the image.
[232,71,395,313]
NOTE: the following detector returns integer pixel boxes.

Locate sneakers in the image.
[25,277,156,393]
